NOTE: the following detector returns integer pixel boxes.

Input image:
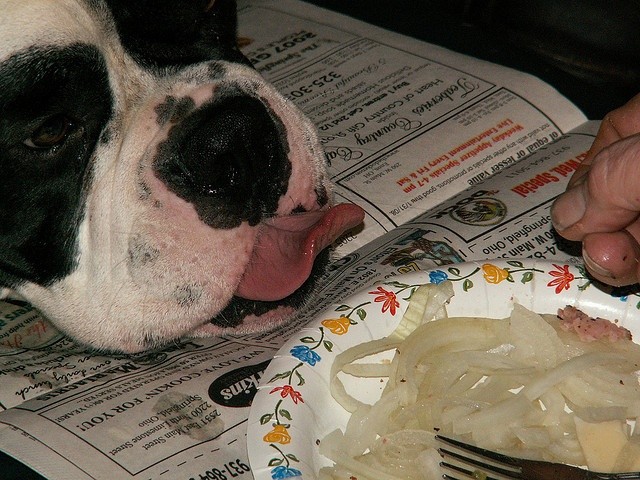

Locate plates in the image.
[248,256,640,480]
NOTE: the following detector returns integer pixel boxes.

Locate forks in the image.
[435,435,640,479]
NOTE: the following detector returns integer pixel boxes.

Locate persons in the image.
[550,99,640,288]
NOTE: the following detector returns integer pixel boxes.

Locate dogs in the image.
[0,0,370,360]
